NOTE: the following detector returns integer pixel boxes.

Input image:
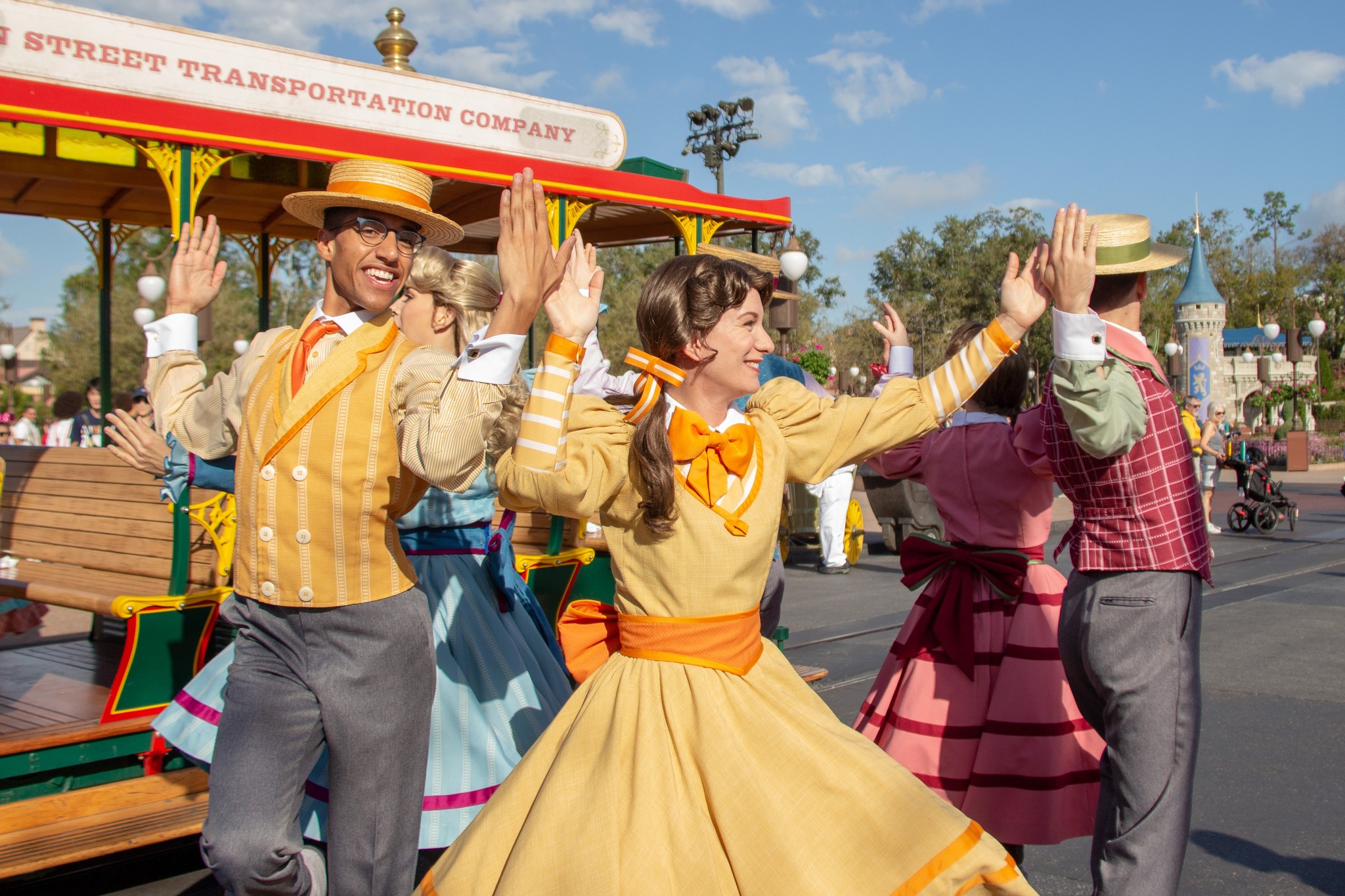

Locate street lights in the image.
[827,360,868,398]
[1166,322,1185,394]
[1262,295,1325,432]
[770,226,808,361]
[681,97,763,198]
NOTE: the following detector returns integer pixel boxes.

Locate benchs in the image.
[491,498,595,643]
[2,445,236,723]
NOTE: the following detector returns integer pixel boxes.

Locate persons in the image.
[814,463,851,574]
[146,157,579,895]
[0,376,163,643]
[1040,201,1220,896]
[570,228,895,682]
[406,227,1057,895]
[1201,401,1229,534]
[103,245,587,853]
[852,300,1111,876]
[1180,395,1203,498]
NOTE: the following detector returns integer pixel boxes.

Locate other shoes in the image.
[299,844,328,896]
[815,560,850,574]
[787,660,829,682]
[1206,522,1222,535]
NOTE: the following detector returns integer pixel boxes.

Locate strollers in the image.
[1220,454,1297,533]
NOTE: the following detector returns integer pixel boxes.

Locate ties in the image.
[289,321,341,401]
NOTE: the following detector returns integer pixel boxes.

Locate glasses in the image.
[134,398,150,404]
[0,432,11,436]
[327,216,428,256]
[1189,404,1202,408]
[1215,412,1225,415]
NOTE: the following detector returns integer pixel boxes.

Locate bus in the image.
[3,4,795,886]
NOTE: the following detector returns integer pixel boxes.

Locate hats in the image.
[0,412,14,425]
[280,158,466,247]
[1025,213,1190,277]
[132,386,148,399]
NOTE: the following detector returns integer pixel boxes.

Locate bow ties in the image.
[664,406,756,509]
